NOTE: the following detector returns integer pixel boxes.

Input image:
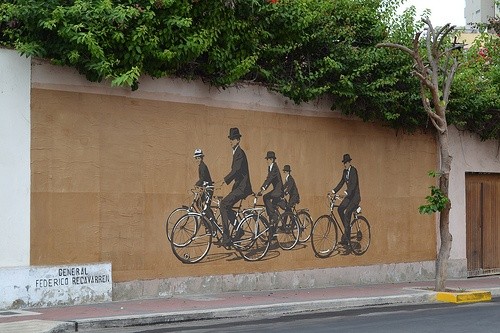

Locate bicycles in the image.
[267,192,314,243]
[230,191,299,252]
[311,191,371,258]
[170,180,270,264]
[166,185,244,248]
